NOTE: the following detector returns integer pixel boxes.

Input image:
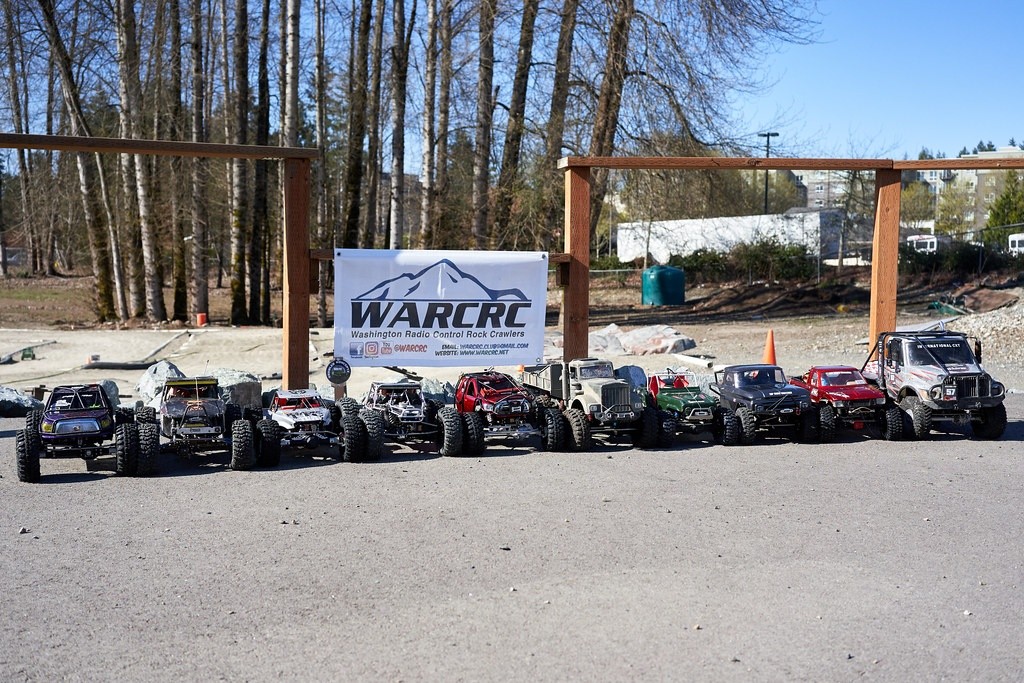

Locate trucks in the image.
[520,358,660,453]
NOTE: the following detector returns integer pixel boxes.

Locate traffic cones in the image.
[760,329,777,366]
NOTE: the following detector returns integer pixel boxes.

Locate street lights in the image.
[758,132,779,213]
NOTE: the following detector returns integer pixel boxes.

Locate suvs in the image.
[860,329,1010,441]
[333,380,464,462]
[708,364,812,447]
[790,365,903,446]
[132,377,259,478]
[453,366,567,458]
[636,368,739,449]
[242,388,365,470]
[14,384,139,483]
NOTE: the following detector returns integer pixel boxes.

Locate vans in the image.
[1008,233,1024,258]
[905,235,952,256]
